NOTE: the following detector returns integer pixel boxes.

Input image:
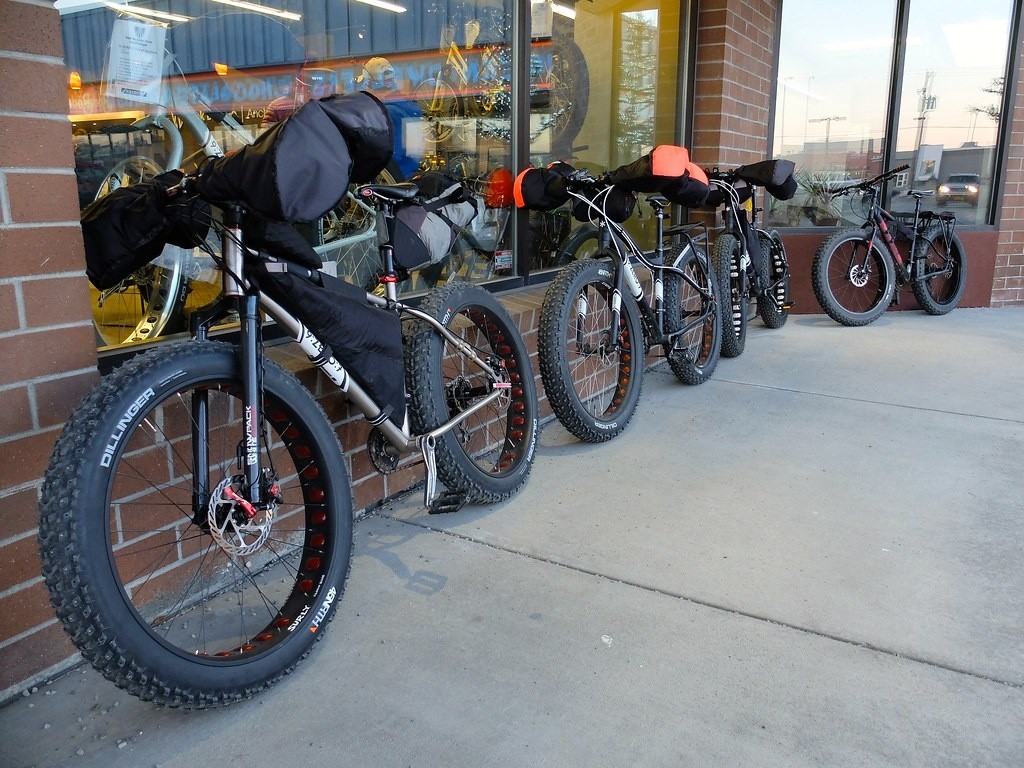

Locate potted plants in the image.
[766,160,852,227]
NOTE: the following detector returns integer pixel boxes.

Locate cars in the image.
[936,173,987,201]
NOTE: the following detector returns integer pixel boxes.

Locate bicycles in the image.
[809,161,970,323]
[712,159,804,354]
[485,144,730,444]
[36,93,547,716]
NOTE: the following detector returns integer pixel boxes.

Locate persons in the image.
[358,58,423,178]
[260,59,317,136]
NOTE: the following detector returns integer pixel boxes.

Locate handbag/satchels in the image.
[384,176,478,272]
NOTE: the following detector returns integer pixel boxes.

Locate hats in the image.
[357,57,395,83]
